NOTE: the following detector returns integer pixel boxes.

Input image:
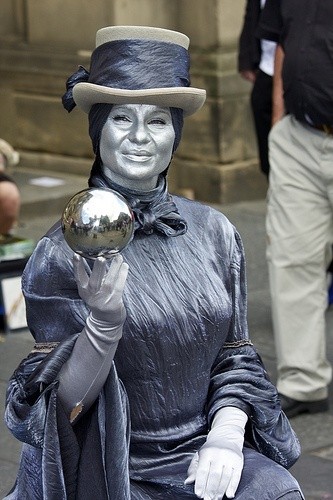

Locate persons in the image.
[240,7,278,183]
[248,1,333,421]
[2,26,307,500]
[0,137,25,243]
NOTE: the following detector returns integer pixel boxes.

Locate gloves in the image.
[56,252,129,424]
[184,406,248,500]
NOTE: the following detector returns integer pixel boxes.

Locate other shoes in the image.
[279,392,328,419]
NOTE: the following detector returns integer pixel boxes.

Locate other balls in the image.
[60,188,133,258]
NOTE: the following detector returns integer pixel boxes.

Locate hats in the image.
[62,26,206,118]
